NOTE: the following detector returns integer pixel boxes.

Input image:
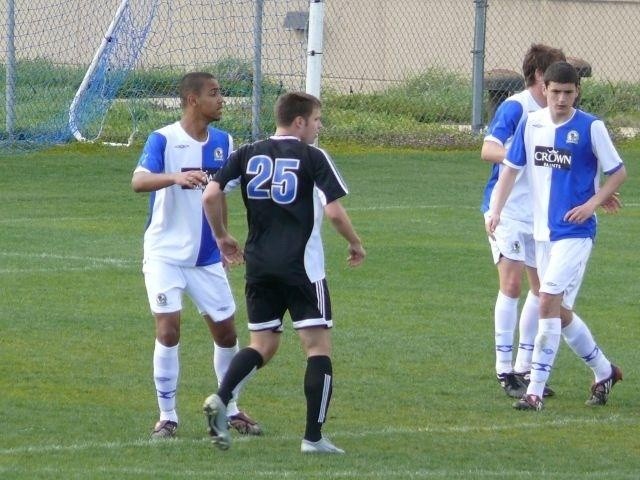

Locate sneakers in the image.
[226,411,263,435]
[511,394,544,413]
[584,363,623,407]
[149,420,178,443]
[201,393,233,450]
[300,435,347,454]
[496,371,554,399]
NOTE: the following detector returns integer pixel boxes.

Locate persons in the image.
[201,91,367,455]
[130,73,261,440]
